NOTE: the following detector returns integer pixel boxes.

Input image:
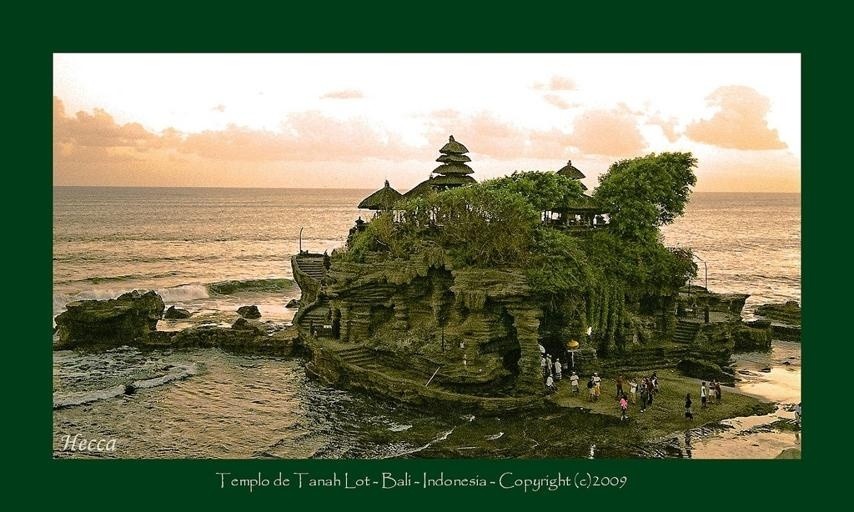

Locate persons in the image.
[539,344,723,423]
